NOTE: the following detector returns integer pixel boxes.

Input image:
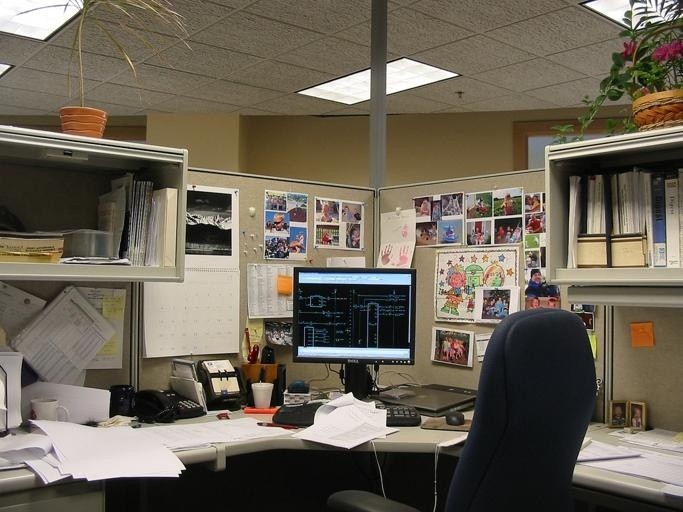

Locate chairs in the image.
[326,306,598,512]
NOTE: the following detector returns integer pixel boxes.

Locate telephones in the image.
[132,389,207,427]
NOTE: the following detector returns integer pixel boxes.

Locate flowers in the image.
[553,0,683,143]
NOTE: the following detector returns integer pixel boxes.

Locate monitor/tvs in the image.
[292,267,416,404]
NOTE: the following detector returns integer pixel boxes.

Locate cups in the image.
[30,397,70,422]
[252,382,274,409]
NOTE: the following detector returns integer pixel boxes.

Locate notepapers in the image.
[241,316,264,343]
[241,343,261,361]
[101,296,125,322]
[629,321,654,347]
[97,333,118,354]
[277,274,293,296]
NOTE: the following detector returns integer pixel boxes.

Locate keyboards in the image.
[272,398,421,426]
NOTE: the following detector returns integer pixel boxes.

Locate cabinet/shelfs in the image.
[1,125,188,283]
[544,127,683,306]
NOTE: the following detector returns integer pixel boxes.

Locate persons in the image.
[527,215,542,232]
[354,209,360,219]
[439,335,465,363]
[633,408,641,427]
[526,196,540,212]
[269,213,286,231]
[473,227,484,244]
[468,197,490,216]
[501,194,516,213]
[485,294,508,317]
[526,298,538,307]
[266,194,286,210]
[548,298,557,306]
[613,407,624,423]
[321,232,332,244]
[290,232,305,254]
[530,270,542,286]
[321,204,332,221]
[497,222,522,243]
[342,205,352,218]
[265,237,288,258]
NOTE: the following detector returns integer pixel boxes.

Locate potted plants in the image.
[17,0,192,142]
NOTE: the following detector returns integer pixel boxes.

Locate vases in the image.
[632,88,683,130]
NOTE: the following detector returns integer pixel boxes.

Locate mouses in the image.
[446,411,464,425]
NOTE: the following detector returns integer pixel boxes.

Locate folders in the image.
[577,174,608,267]
[610,170,645,266]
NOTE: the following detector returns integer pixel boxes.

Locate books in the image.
[567,159,683,268]
[95,170,177,268]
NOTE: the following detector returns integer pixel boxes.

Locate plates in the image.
[245,407,277,413]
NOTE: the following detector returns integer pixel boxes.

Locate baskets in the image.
[632,25,683,131]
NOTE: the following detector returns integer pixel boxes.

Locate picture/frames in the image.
[609,401,626,427]
[629,402,646,429]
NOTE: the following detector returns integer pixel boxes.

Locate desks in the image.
[0,384,683,512]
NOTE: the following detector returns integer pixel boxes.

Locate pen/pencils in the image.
[576,454,640,461]
[257,422,298,429]
